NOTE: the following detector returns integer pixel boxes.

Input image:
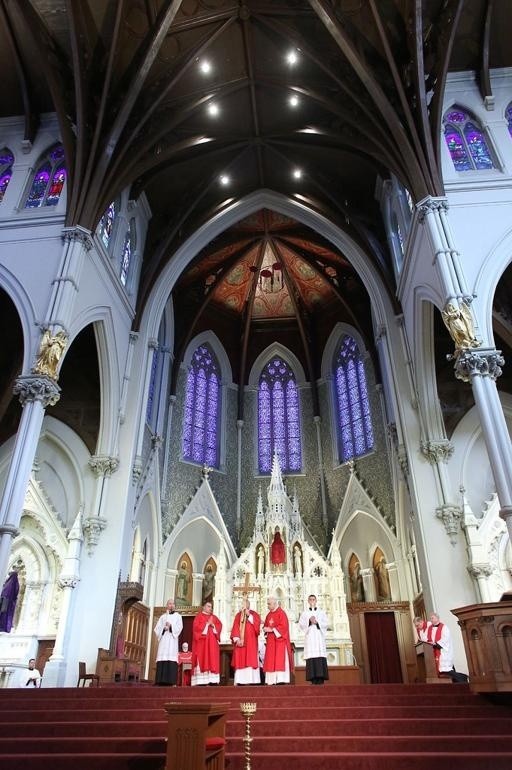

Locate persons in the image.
[202,564,215,601]
[0,558,26,633]
[427,611,455,672]
[177,641,193,687]
[348,561,365,602]
[257,545,265,574]
[228,598,262,686]
[298,594,329,684]
[294,545,302,574]
[263,596,295,686]
[374,554,391,602]
[191,600,223,686]
[21,658,42,688]
[153,598,185,685]
[440,302,473,347]
[411,615,433,643]
[175,560,192,600]
[35,329,67,380]
[271,524,286,565]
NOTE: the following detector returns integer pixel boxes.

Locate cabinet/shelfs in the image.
[164,701,233,770]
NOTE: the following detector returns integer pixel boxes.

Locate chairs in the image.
[77,662,100,688]
